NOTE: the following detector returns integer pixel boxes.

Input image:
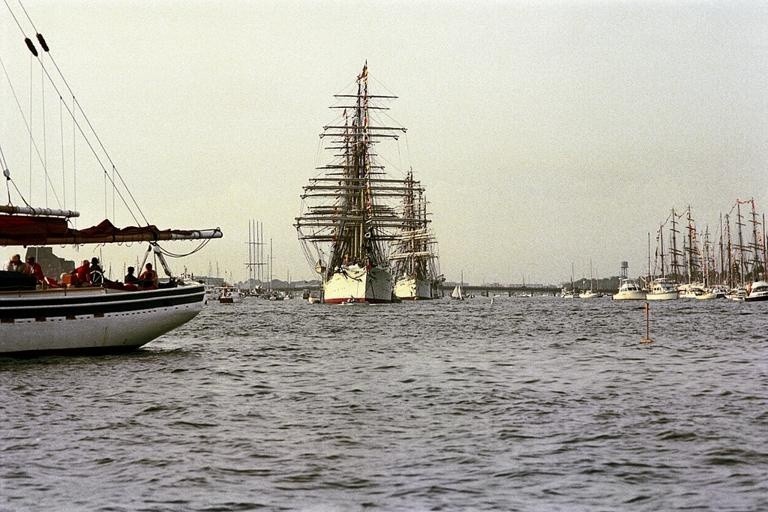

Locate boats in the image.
[561,288,603,299]
[204,284,245,304]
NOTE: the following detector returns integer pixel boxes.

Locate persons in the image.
[2,255,16,271]
[24,255,45,284]
[69,259,93,286]
[13,253,26,272]
[89,257,118,287]
[125,266,142,286]
[138,263,159,289]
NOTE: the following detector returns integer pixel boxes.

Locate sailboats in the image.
[1,2,222,354]
[448,284,464,301]
[612,196,768,302]
[292,57,446,305]
